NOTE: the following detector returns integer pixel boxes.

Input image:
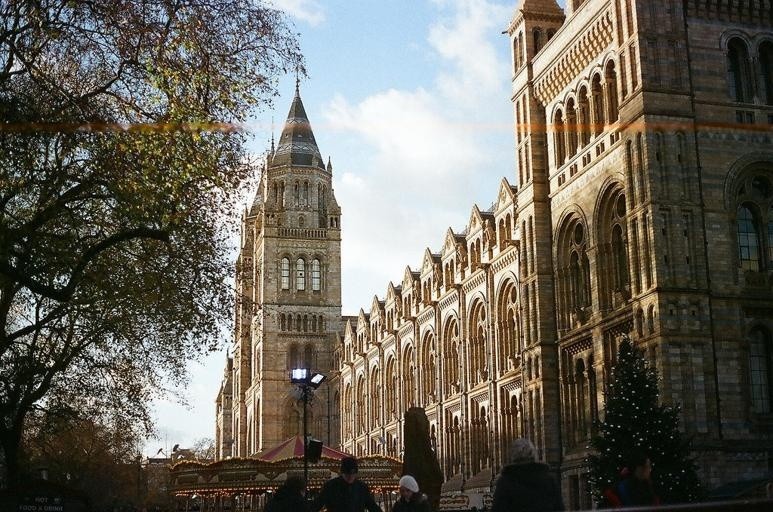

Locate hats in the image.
[399,474,419,493]
[511,438,536,462]
[340,457,359,475]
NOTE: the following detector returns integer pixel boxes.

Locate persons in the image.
[590,444,663,512]
[489,436,565,512]
[259,476,327,512]
[388,475,434,512]
[318,456,383,512]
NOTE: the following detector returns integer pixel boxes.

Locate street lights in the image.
[290,368,327,500]
